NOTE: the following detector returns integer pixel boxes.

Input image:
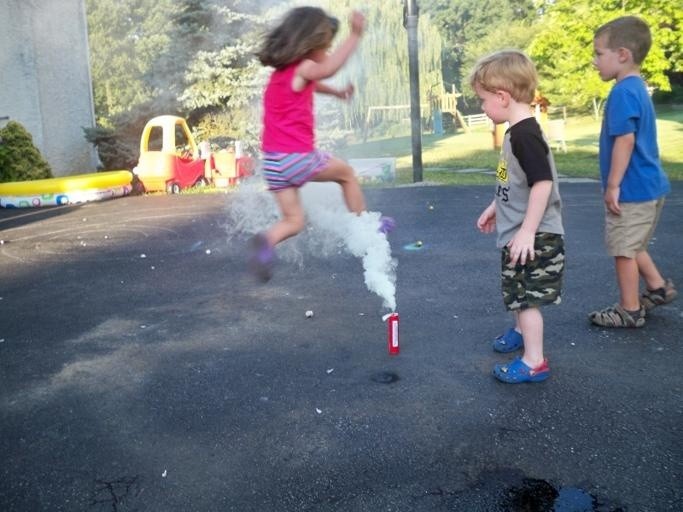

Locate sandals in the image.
[493,356,550,384]
[493,327,524,354]
[640,278,678,311]
[588,300,648,328]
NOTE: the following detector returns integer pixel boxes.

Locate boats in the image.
[1,170,133,209]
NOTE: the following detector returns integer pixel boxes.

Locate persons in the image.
[586,16,677,328]
[471,51,564,384]
[249,6,393,269]
